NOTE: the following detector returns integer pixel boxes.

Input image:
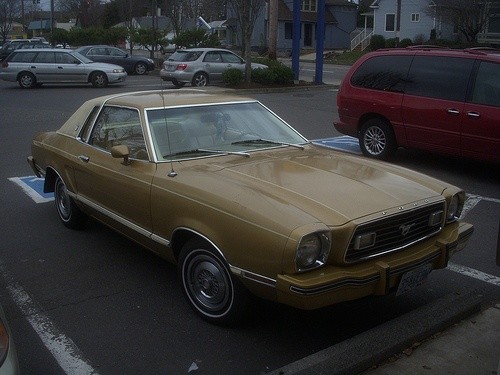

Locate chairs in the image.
[95,122,224,156]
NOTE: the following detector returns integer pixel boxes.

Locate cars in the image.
[159,46,270,89]
[26,86,475,325]
[0,36,73,59]
[0,48,128,89]
[63,44,155,75]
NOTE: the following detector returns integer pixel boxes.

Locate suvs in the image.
[333,44,500,164]
